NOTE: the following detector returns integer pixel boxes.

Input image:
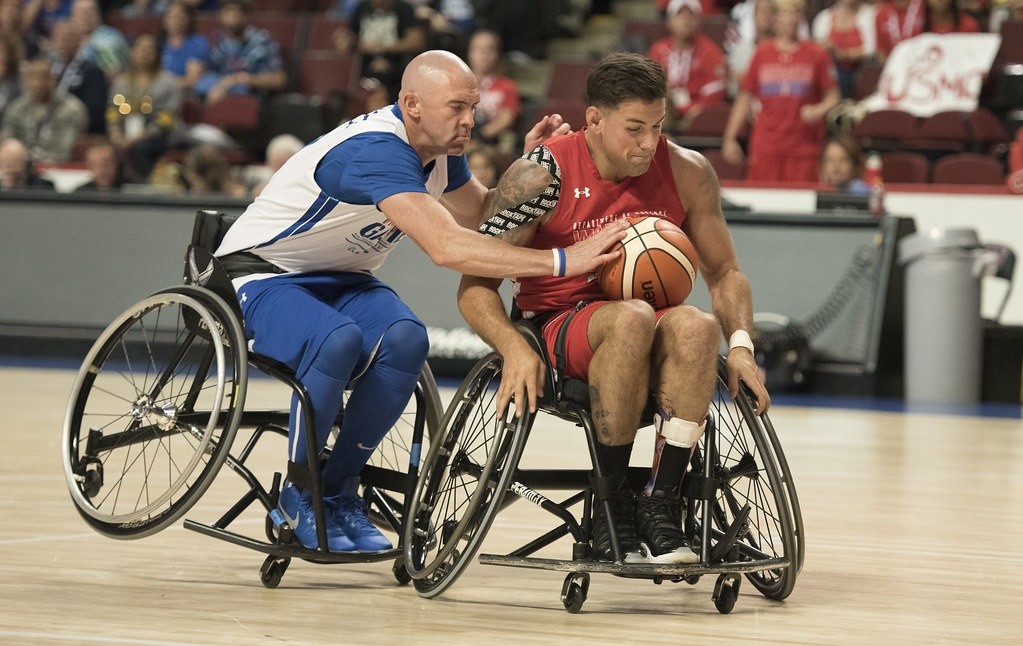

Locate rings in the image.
[603,228,607,231]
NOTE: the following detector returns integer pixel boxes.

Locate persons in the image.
[0,1,309,197]
[215,51,630,552]
[647,1,1023,188]
[819,134,871,195]
[457,55,771,563]
[335,0,634,191]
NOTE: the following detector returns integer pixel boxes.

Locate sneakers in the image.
[277,478,356,552]
[636,494,699,564]
[592,490,649,564]
[321,489,393,551]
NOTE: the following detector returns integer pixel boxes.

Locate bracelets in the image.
[558,247,566,277]
[552,248,560,277]
[728,329,754,357]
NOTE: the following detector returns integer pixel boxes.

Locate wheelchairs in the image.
[400,302,806,614]
[62,210,447,587]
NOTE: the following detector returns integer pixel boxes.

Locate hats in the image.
[666,0,703,16]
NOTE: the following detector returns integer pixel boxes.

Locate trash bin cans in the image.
[896,229,983,405]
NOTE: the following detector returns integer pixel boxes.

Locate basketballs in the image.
[597,217,699,310]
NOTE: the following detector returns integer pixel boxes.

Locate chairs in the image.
[110,15,360,135]
[527,5,1022,186]
